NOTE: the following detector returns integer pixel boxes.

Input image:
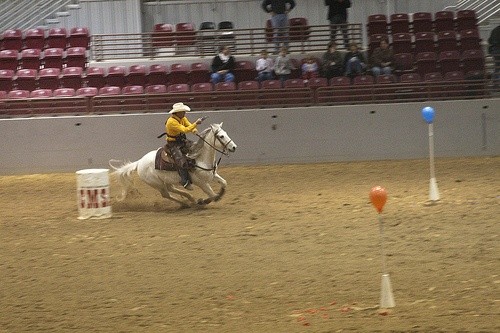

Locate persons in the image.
[325,0,352,51]
[370,38,394,77]
[322,42,344,79]
[488,26,500,91]
[165,103,202,188]
[344,43,366,81]
[209,46,235,84]
[256,50,273,81]
[302,56,319,83]
[274,47,291,87]
[261,0,296,55]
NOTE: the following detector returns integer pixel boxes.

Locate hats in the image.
[169,103,190,114]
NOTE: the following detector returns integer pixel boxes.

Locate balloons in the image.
[369,186,387,211]
[422,106,435,123]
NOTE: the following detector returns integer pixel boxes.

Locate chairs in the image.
[0,9,486,117]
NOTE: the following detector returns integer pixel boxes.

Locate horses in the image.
[109,122,237,209]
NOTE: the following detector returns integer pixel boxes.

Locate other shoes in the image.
[182,179,189,189]
